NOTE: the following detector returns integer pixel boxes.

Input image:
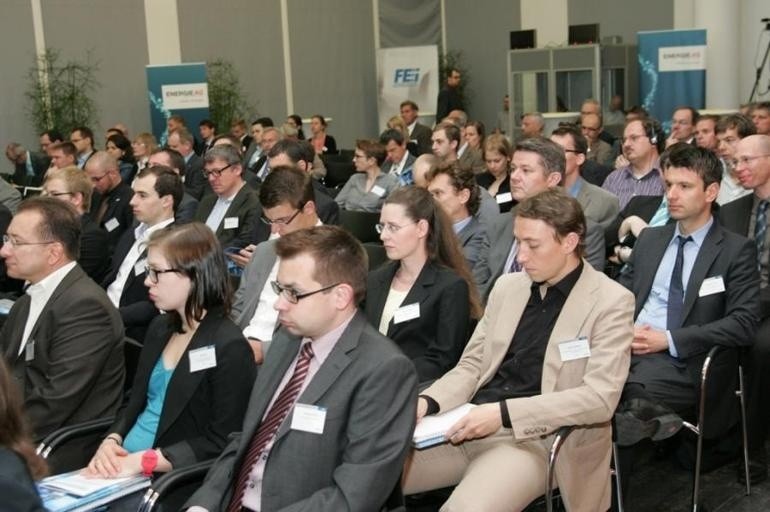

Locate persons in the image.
[436,68,465,126]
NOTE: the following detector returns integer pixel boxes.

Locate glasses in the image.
[375,222,417,234]
[270,279,338,305]
[260,208,302,225]
[203,163,232,179]
[726,157,765,165]
[3,232,53,247]
[355,153,370,158]
[3,128,126,198]
[582,125,601,130]
[143,265,184,284]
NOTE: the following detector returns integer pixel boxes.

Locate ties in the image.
[95,196,110,228]
[752,200,769,274]
[509,252,524,274]
[260,159,271,182]
[666,234,695,343]
[227,342,316,511]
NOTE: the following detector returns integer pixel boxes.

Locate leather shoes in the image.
[734,446,768,490]
[611,398,685,448]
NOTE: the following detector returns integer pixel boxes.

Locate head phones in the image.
[651,120,660,144]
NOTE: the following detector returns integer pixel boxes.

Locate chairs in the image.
[546,413,622,511]
[680,344,750,511]
